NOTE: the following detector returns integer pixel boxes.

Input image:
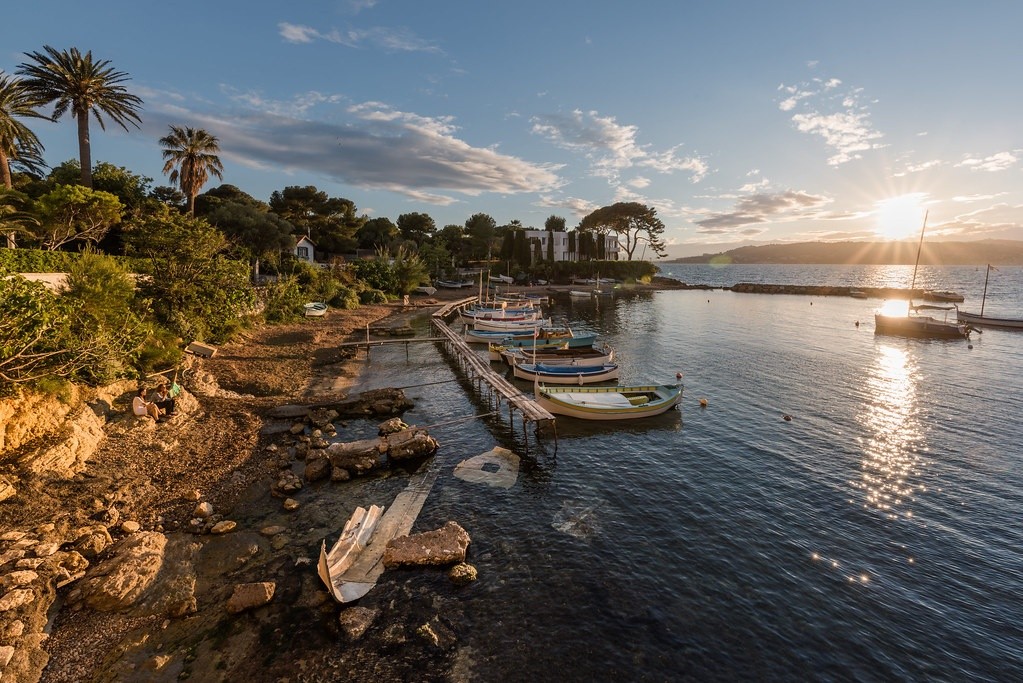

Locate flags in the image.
[990,266,999,272]
[500,275,513,283]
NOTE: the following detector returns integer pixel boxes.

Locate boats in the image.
[850,290,868,298]
[874,209,972,337]
[488,273,515,284]
[459,292,614,369]
[571,277,616,284]
[953,262,1023,326]
[533,373,685,420]
[297,301,328,317]
[435,279,475,288]
[923,289,964,303]
[513,352,619,386]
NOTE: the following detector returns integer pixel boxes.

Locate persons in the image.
[151,384,177,417]
[133,388,167,423]
